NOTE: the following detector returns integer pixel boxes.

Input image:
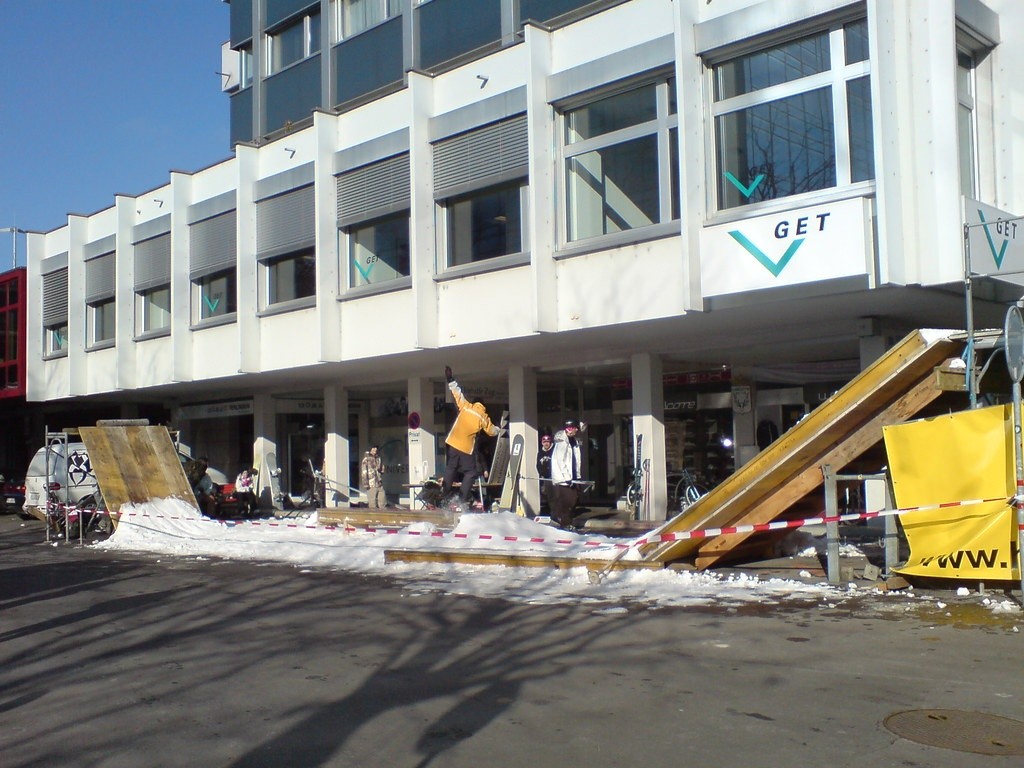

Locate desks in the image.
[401,481,503,511]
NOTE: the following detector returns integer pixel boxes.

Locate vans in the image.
[23,440,189,525]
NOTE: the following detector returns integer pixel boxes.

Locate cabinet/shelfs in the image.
[666,418,718,471]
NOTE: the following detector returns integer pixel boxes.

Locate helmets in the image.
[542,435,551,443]
[564,418,578,427]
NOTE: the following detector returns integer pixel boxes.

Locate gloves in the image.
[566,481,573,487]
[445,366,455,383]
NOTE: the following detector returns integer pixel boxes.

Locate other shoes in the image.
[562,524,577,531]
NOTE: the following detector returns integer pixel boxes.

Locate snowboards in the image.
[264,451,286,514]
[495,435,525,514]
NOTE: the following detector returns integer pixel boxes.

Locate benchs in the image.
[217,483,237,519]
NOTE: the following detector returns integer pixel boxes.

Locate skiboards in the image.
[634,433,641,521]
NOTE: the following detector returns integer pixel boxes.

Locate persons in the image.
[236,469,256,518]
[361,441,388,509]
[443,365,502,510]
[190,456,215,516]
[537,417,582,531]
